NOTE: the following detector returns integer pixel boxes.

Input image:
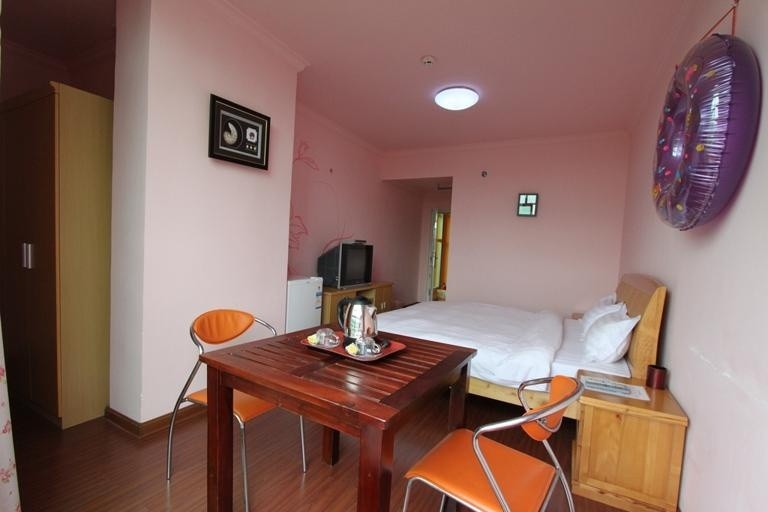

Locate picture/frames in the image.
[206,94,271,170]
[516,193,538,216]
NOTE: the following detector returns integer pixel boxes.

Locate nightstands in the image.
[570,369,689,511]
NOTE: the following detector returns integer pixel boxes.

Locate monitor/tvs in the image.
[317,242,373,291]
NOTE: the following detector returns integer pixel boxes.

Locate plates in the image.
[310,341,339,348]
[347,349,385,357]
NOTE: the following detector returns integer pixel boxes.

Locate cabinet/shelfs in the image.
[0,80,114,432]
[321,280,393,325]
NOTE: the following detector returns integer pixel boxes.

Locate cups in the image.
[645,364,667,389]
[355,336,381,353]
[316,328,340,345]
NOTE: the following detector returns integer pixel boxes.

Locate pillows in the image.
[576,292,642,364]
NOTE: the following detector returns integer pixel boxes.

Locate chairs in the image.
[399,375,584,511]
[165,309,307,512]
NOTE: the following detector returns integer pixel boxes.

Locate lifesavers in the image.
[650,32,762,232]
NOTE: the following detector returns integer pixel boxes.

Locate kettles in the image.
[336,296,379,344]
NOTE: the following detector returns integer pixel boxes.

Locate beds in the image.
[376,271,667,420]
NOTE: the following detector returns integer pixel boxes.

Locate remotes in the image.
[584,378,631,395]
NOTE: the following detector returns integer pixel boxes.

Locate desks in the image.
[199,323,478,512]
